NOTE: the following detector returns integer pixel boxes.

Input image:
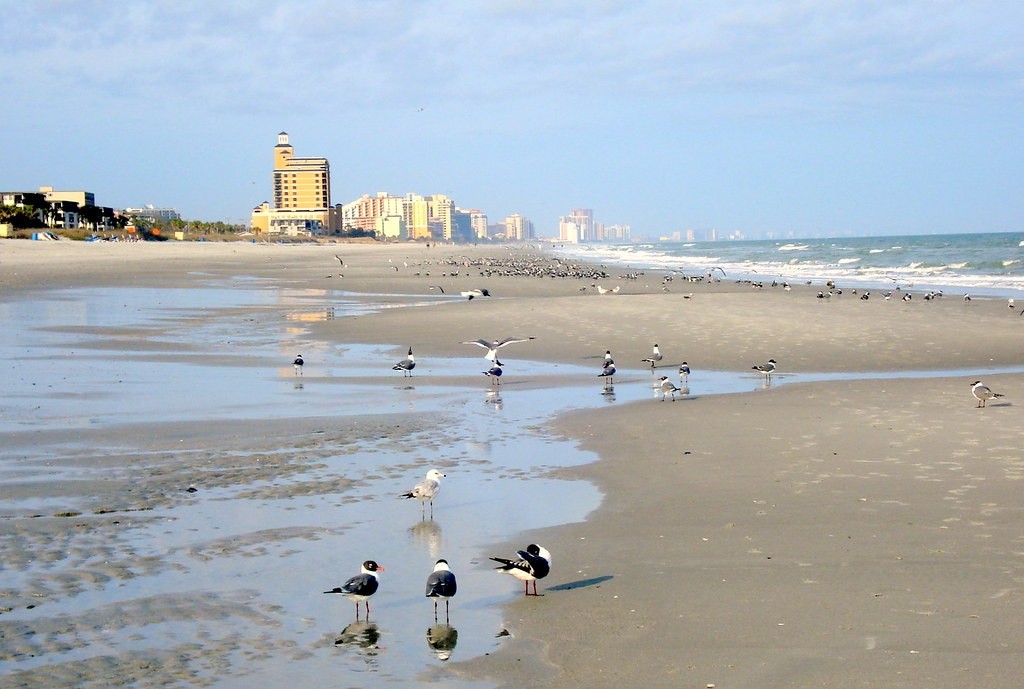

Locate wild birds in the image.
[323,255,944,302]
[1007,298,1016,312]
[488,544,552,596]
[397,469,447,518]
[963,292,971,306]
[640,343,690,402]
[391,346,416,378]
[751,358,777,379]
[1018,307,1024,318]
[291,354,305,374]
[407,519,442,558]
[426,613,459,662]
[458,335,539,386]
[970,380,1005,408]
[597,351,617,384]
[425,558,457,613]
[331,612,381,657]
[323,560,385,612]
